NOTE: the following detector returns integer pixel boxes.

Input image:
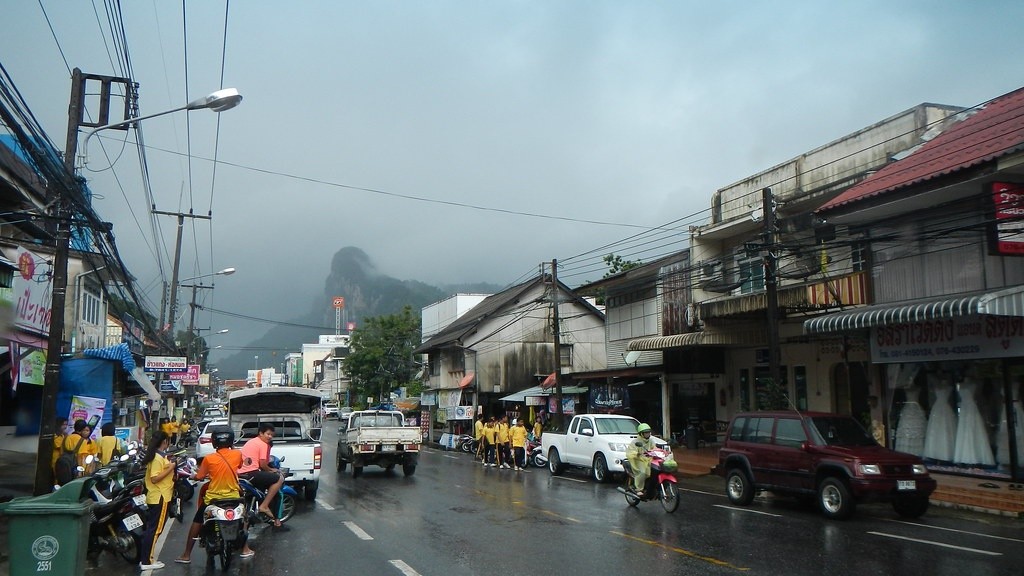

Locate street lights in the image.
[166,267,234,344]
[34,87,243,490]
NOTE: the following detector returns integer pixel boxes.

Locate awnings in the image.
[803,284,1024,335]
[626,327,767,349]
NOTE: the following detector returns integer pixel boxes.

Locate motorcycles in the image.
[238,455,298,527]
[616,442,681,513]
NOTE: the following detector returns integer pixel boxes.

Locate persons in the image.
[138,430,176,570]
[474,414,527,471]
[533,416,543,438]
[53,417,122,484]
[625,423,658,496]
[174,426,255,563]
[237,422,283,526]
[161,416,179,451]
[180,419,190,436]
[892,376,1024,469]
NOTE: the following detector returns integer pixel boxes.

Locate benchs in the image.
[703,420,730,448]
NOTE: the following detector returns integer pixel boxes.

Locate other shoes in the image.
[500,465,504,468]
[491,463,497,467]
[139,559,165,570]
[258,508,274,519]
[475,457,481,460]
[504,462,510,468]
[637,491,644,495]
[519,467,523,471]
[273,525,289,532]
[484,463,488,466]
[515,465,519,471]
[482,459,484,464]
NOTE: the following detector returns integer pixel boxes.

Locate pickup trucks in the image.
[542,413,673,484]
[227,386,325,502]
[335,410,423,480]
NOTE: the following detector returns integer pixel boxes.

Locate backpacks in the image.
[55,437,84,484]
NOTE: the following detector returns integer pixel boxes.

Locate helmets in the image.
[211,425,234,448]
[638,423,651,434]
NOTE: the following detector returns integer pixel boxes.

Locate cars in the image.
[322,404,339,418]
[337,407,353,422]
[195,422,228,465]
[194,401,228,439]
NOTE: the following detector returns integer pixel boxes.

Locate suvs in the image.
[709,408,938,522]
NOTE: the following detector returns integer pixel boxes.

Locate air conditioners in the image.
[697,260,723,282]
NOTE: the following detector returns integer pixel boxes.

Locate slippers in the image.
[175,555,191,563]
[240,549,255,557]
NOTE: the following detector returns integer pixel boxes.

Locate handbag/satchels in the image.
[110,438,121,461]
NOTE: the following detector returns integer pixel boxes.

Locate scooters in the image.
[459,433,548,468]
[53,427,202,566]
[199,476,256,573]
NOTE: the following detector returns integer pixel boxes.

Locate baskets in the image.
[280,467,289,478]
[661,461,679,473]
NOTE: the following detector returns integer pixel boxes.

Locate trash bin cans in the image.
[0,477,100,576]
[685,427,698,449]
